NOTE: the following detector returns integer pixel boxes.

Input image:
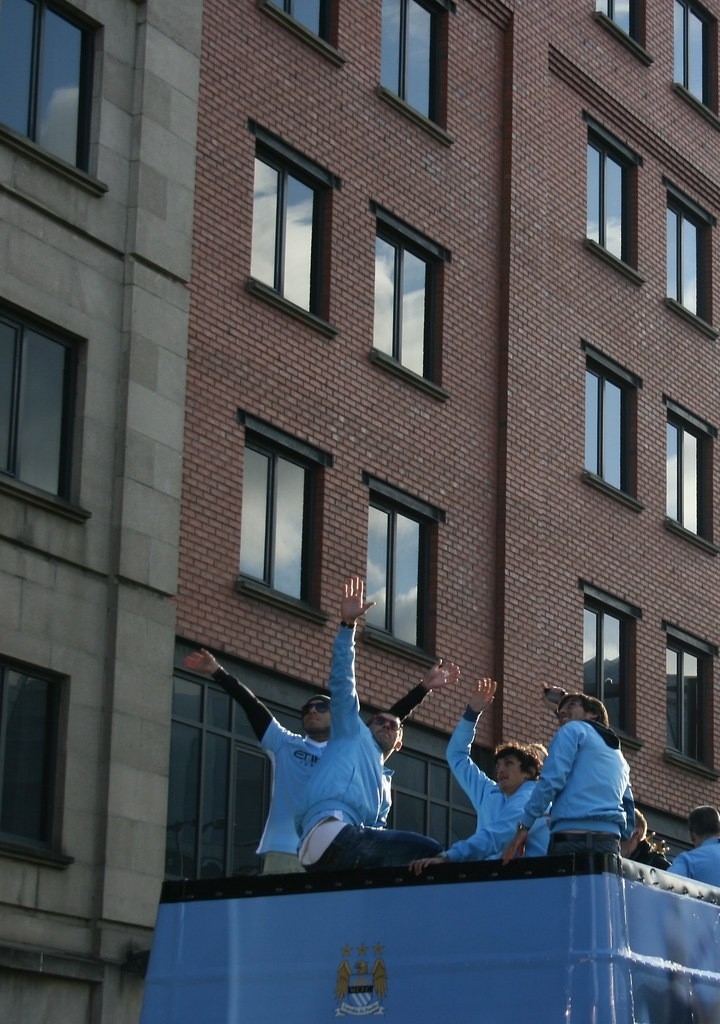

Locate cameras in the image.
[542,686,566,703]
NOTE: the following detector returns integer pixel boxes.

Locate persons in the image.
[294,578,441,872]
[623,809,672,870]
[183,647,460,877]
[667,805,720,887]
[409,677,551,874]
[527,743,548,763]
[499,693,634,863]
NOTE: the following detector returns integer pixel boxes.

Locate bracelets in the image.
[340,621,358,628]
[212,667,224,676]
[518,824,530,831]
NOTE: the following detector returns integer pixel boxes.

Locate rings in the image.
[417,860,420,862]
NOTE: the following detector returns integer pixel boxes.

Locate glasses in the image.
[301,702,330,713]
[371,714,404,731]
